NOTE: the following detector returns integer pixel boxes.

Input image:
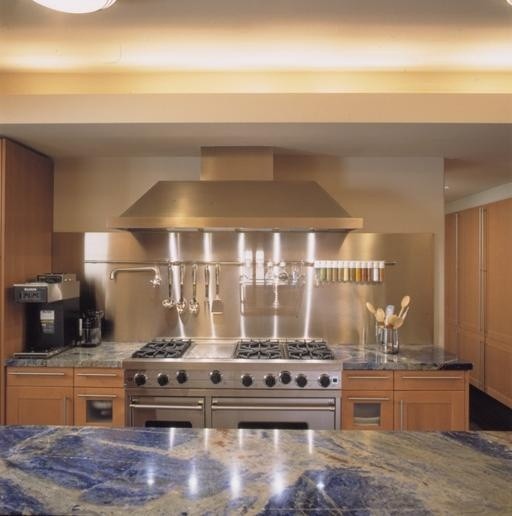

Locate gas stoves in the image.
[124,338,342,388]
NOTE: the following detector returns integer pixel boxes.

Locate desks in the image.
[1,424,512,516]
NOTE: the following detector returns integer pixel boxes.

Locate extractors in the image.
[109,147,364,231]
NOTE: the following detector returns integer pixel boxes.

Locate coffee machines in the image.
[12,271,81,359]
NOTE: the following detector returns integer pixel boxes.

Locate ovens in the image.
[129,398,335,430]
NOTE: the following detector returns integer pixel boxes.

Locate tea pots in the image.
[73,307,105,347]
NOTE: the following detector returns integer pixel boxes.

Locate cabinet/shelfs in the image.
[6,366,74,426]
[393,371,469,432]
[341,370,393,431]
[74,367,125,428]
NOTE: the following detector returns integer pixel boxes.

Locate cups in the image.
[377,322,399,355]
